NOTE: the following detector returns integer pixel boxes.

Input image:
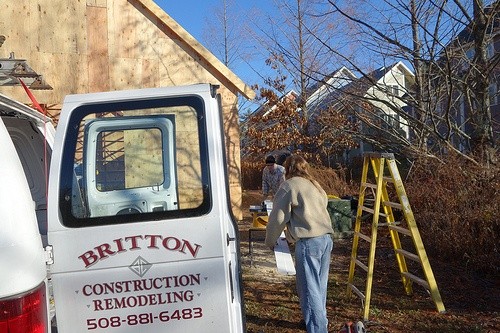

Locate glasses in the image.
[265,164,274,166]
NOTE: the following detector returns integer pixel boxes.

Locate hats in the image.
[265,155,276,164]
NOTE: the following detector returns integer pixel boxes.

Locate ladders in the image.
[346,150,447,321]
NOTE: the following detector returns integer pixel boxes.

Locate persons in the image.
[262,156,287,201]
[265,156,335,333]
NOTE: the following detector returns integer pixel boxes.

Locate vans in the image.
[1,35,245,333]
